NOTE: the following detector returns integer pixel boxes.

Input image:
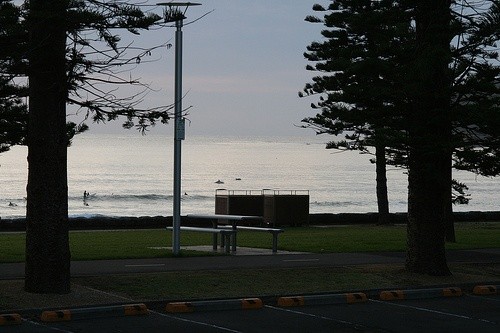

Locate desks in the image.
[186,212,262,252]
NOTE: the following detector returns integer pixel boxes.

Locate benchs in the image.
[167,224,235,252]
[217,224,283,254]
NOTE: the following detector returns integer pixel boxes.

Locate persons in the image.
[8,202,13,206]
[83,191,87,198]
